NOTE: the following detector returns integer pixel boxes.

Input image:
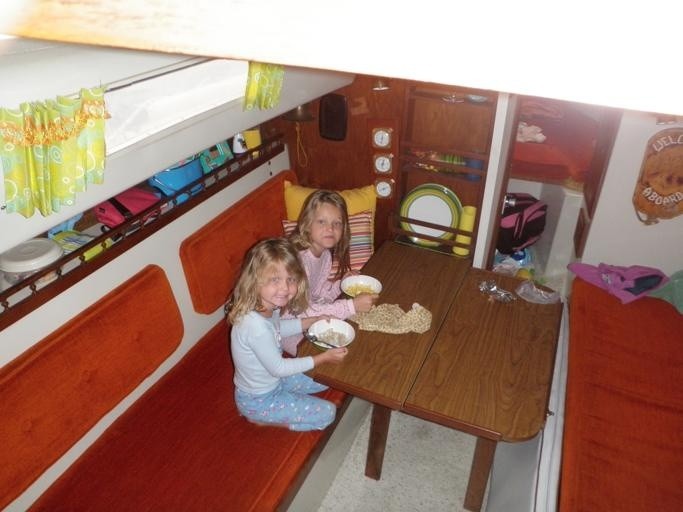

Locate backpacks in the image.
[92,186,161,231]
[497,191,548,253]
[146,156,206,207]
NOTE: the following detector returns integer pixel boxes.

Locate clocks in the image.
[372,127,395,200]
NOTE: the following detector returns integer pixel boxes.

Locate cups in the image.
[464,157,484,181]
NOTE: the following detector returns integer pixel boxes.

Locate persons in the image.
[226,188,379,433]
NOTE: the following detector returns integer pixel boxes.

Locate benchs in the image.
[482,269,682,512]
[0,169,374,511]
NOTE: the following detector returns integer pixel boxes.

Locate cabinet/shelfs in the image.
[390,81,498,264]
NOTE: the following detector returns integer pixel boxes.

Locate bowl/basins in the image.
[1,238,65,285]
[307,317,357,350]
[399,183,463,247]
[340,275,383,299]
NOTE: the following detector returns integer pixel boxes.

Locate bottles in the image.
[505,197,517,210]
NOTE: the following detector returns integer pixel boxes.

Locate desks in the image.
[293,242,562,512]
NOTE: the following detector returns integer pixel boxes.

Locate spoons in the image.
[304,332,340,348]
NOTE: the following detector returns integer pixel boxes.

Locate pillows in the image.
[281,179,376,275]
